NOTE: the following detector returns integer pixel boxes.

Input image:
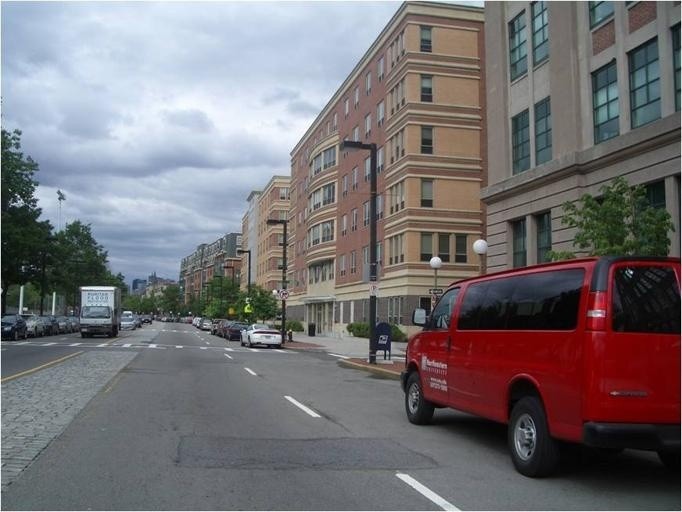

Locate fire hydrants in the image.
[287,332,293,343]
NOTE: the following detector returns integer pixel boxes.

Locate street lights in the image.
[202,248,250,298]
[429,256,443,293]
[473,240,489,275]
[337,139,377,367]
[267,218,287,343]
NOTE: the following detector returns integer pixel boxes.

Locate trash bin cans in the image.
[308,322,316,336]
[375,322,391,360]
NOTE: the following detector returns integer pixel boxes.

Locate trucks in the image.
[78,286,121,336]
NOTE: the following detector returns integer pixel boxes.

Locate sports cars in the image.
[239,323,282,348]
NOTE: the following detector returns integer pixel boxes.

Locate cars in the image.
[1,313,80,341]
[156,315,193,323]
[121,311,151,329]
[193,317,248,340]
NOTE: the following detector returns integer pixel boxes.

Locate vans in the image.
[401,255,681,478]
